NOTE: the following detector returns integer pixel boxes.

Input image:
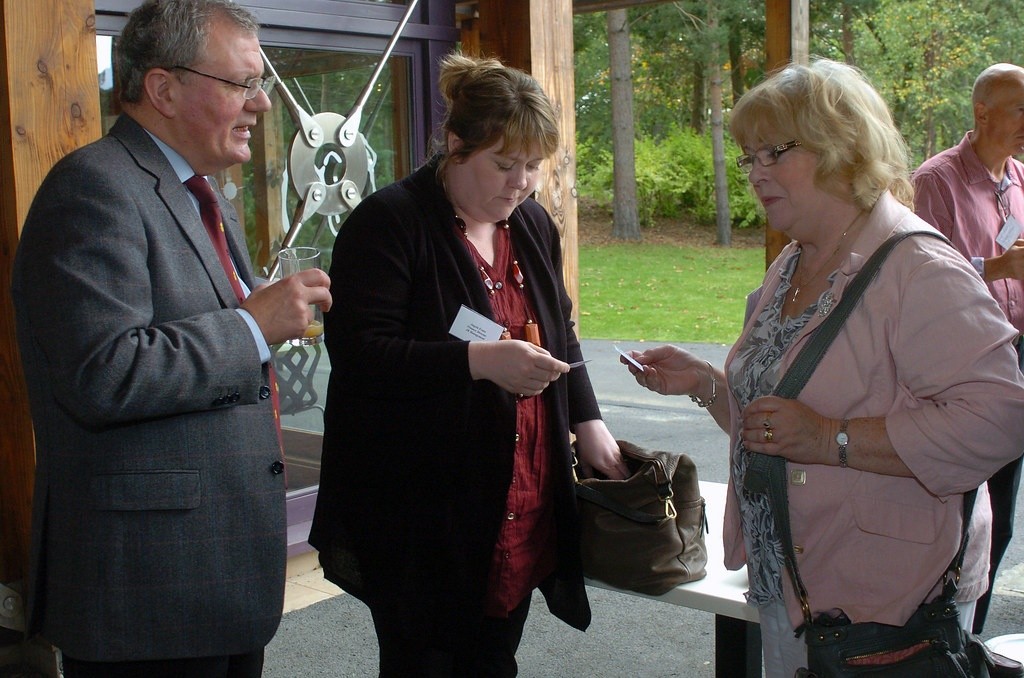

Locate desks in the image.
[581,479,764,678]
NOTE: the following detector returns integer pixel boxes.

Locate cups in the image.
[277,247,323,346]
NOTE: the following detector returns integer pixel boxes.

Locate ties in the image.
[188,173,287,484]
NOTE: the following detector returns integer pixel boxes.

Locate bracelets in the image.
[970,257,984,279]
[690,361,717,408]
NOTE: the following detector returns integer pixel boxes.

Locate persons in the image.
[912,63,1024,678]
[623,59,1024,678]
[8,0,332,678]
[306,56,627,678]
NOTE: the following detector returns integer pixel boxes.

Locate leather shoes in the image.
[976,635,1024,678]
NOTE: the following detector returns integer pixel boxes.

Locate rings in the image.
[763,430,773,443]
[763,412,772,429]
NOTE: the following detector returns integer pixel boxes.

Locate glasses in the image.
[736,136,804,173]
[159,64,277,100]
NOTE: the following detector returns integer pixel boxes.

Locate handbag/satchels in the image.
[799,596,996,678]
[573,438,707,595]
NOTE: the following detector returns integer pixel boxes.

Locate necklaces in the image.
[453,212,540,348]
[792,210,864,301]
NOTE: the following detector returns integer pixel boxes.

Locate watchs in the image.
[835,418,848,468]
